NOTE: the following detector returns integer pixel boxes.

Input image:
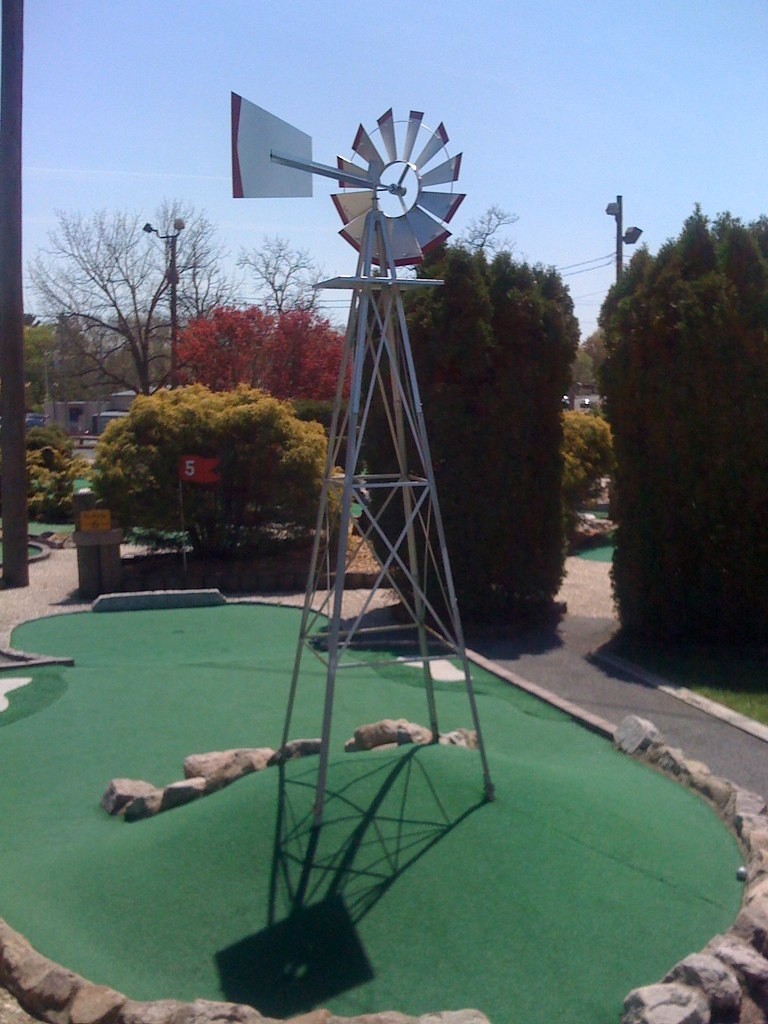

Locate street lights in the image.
[142,219,186,387]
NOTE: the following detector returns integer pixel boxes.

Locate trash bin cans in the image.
[73,528,126,594]
